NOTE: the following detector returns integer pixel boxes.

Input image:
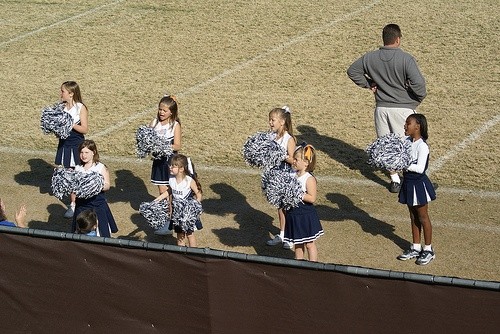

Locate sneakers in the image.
[266,233,295,249]
[397,245,437,265]
[63,203,75,218]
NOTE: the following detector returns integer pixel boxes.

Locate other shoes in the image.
[431,181,438,190]
[391,177,403,192]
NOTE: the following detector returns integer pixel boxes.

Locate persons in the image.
[51,139,118,238]
[262,143,325,262]
[0,197,27,228]
[139,154,204,248]
[75,209,99,237]
[244,106,296,249]
[137,96,181,235]
[347,24,438,193]
[367,114,435,265]
[41,81,88,218]
